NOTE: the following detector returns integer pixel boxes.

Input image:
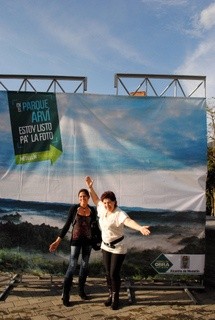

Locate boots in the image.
[61,279,70,307]
[104,288,113,306]
[111,291,119,310]
[78,276,88,300]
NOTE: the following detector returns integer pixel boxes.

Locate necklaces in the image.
[81,205,88,213]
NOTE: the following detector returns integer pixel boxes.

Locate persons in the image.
[84,176,151,310]
[50,189,102,307]
